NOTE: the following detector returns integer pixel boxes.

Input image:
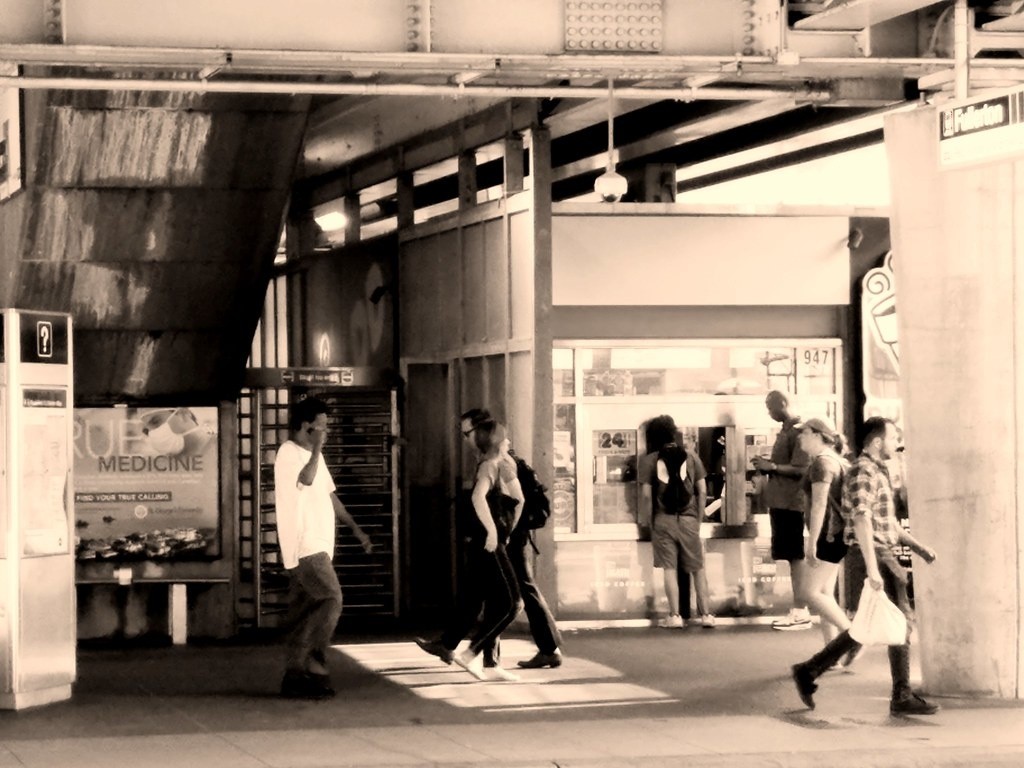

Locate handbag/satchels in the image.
[849,578,906,646]
[460,460,520,545]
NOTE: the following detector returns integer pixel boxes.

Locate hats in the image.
[475,418,496,454]
[793,417,836,436]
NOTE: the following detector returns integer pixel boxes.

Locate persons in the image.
[273,397,373,699]
[415,409,565,680]
[795,418,867,669]
[636,415,715,629]
[751,391,813,632]
[791,416,941,715]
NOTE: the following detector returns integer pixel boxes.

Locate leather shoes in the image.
[518,647,562,668]
[412,637,453,665]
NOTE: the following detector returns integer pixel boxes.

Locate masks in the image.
[148,408,200,457]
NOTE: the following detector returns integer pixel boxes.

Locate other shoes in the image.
[842,641,866,667]
[792,662,819,710]
[889,692,940,715]
[281,667,336,699]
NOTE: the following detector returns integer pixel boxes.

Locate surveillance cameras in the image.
[595,171,627,202]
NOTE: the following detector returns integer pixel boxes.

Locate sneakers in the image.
[453,647,488,682]
[482,665,519,681]
[772,605,812,631]
[701,613,715,627]
[657,612,684,628]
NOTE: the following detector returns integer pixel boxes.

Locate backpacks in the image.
[651,442,694,515]
[507,449,550,530]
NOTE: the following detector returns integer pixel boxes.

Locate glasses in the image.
[463,426,478,437]
